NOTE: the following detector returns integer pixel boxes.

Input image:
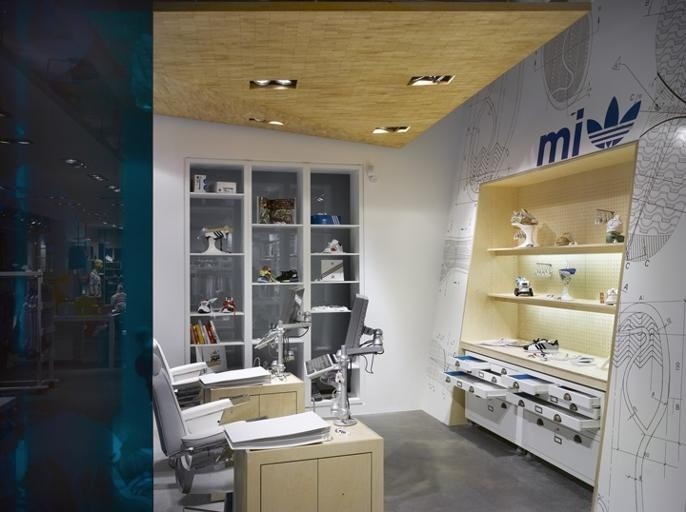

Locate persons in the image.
[88,259,102,299]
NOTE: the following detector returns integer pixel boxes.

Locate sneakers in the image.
[523,338,558,351]
[276,270,298,282]
[323,239,343,253]
[257,265,272,283]
[197,299,212,313]
[221,296,236,312]
[203,228,229,240]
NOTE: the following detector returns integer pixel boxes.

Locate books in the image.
[199,366,270,390]
[223,410,330,450]
[187,319,219,343]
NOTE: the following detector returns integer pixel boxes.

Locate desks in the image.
[51,310,118,370]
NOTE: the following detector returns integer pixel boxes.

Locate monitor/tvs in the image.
[285,288,304,324]
[345,294,368,349]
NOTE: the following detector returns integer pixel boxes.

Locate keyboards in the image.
[253,330,276,350]
[306,354,334,377]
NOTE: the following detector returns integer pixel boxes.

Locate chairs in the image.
[152,354,247,512]
[152,337,207,405]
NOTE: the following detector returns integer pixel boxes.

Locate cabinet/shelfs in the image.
[444,139,638,512]
[232,418,384,512]
[186,158,252,372]
[246,161,309,409]
[204,371,304,425]
[309,162,364,408]
[1,270,59,392]
